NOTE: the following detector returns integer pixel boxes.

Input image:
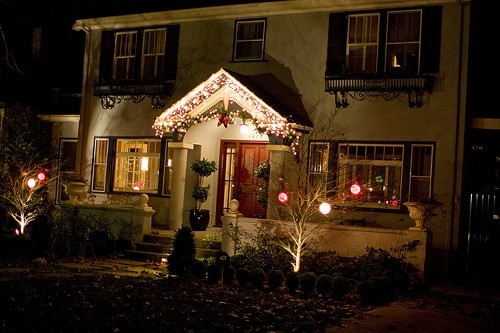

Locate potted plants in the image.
[404,196,443,231]
[189,157,217,230]
[62,176,88,202]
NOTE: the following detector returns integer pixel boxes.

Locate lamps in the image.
[278,193,288,203]
[351,184,361,194]
[319,202,331,215]
[141,158,148,172]
[28,178,35,188]
[38,173,45,180]
